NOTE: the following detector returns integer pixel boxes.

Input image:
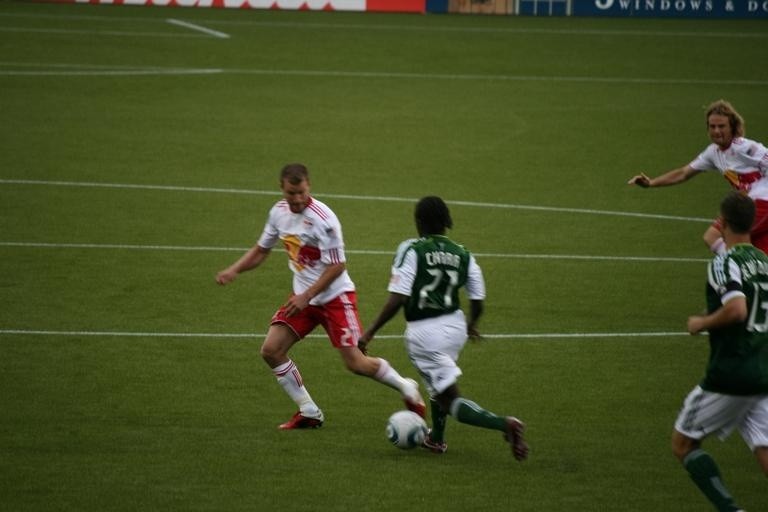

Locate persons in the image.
[358,195,528,462]
[628,100,768,257]
[219,164,426,428]
[670,197,768,512]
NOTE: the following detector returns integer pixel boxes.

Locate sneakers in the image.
[421,433,448,453]
[277,409,324,430]
[403,377,426,418]
[507,416,528,460]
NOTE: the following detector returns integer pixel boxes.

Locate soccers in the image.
[385,411,428,449]
